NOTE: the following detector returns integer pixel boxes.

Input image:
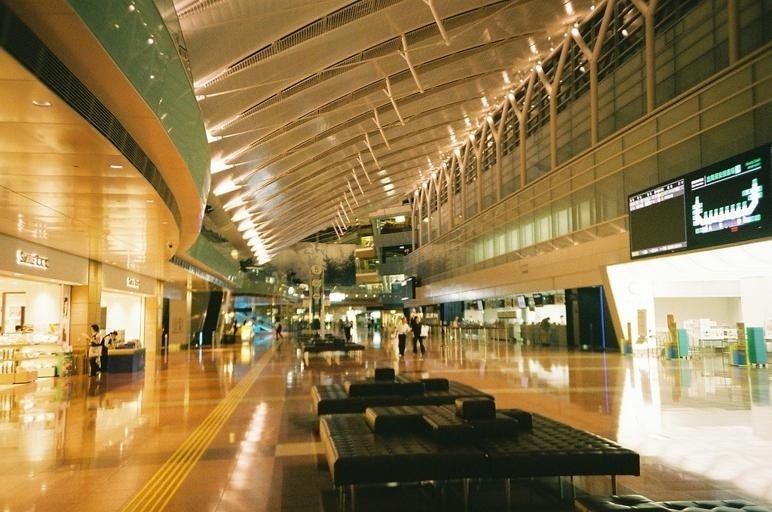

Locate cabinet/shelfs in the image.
[33,342,58,378]
[0,342,39,384]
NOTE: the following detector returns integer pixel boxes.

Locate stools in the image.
[573,494,772,512]
[315,395,639,511]
[307,365,497,419]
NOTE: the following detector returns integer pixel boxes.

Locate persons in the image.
[228,318,238,344]
[338,316,374,342]
[541,317,551,347]
[100,332,118,375]
[559,315,566,325]
[395,316,425,357]
[521,321,536,346]
[85,323,102,378]
[274,317,320,340]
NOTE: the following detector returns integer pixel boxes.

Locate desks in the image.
[699,339,738,354]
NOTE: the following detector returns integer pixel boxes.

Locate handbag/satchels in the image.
[420,325,429,336]
[89,345,103,357]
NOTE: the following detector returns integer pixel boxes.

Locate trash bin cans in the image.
[56,352,73,375]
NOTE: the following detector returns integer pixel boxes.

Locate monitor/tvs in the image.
[476,299,484,311]
[533,294,543,307]
[517,295,527,309]
